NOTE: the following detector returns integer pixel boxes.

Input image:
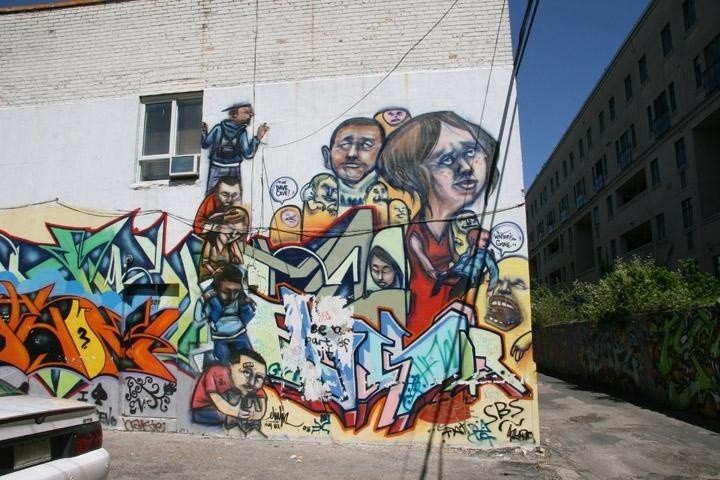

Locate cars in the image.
[0,377,113,479]
